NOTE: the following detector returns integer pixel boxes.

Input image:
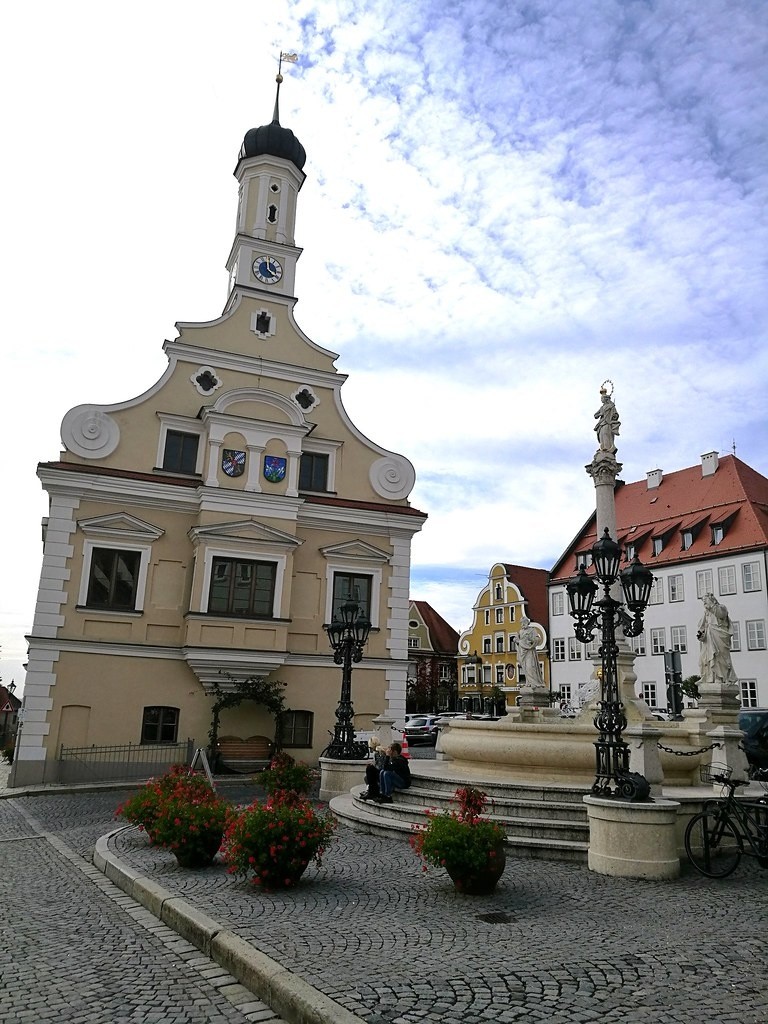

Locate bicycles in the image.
[684,761,768,879]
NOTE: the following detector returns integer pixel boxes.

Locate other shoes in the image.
[373,794,393,803]
[360,793,379,800]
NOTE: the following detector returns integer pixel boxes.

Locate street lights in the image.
[4,680,17,732]
[325,593,365,760]
[566,527,655,803]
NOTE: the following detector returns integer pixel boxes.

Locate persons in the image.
[593,388,620,454]
[512,616,546,687]
[465,709,475,720]
[694,591,738,685]
[358,736,411,803]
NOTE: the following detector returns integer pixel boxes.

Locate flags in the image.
[283,53,298,63]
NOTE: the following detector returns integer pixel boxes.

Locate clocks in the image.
[252,256,283,285]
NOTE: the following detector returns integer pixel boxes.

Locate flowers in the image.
[219,790,338,890]
[113,763,236,855]
[409,788,515,872]
[253,752,315,803]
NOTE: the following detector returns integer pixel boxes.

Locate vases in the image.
[169,830,222,871]
[445,856,507,897]
[252,858,309,887]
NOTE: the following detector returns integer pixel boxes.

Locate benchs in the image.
[217,736,274,768]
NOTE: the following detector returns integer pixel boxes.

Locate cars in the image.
[649,706,685,722]
[738,707,768,766]
[405,714,428,725]
[404,717,442,746]
[652,713,671,721]
[438,712,489,719]
